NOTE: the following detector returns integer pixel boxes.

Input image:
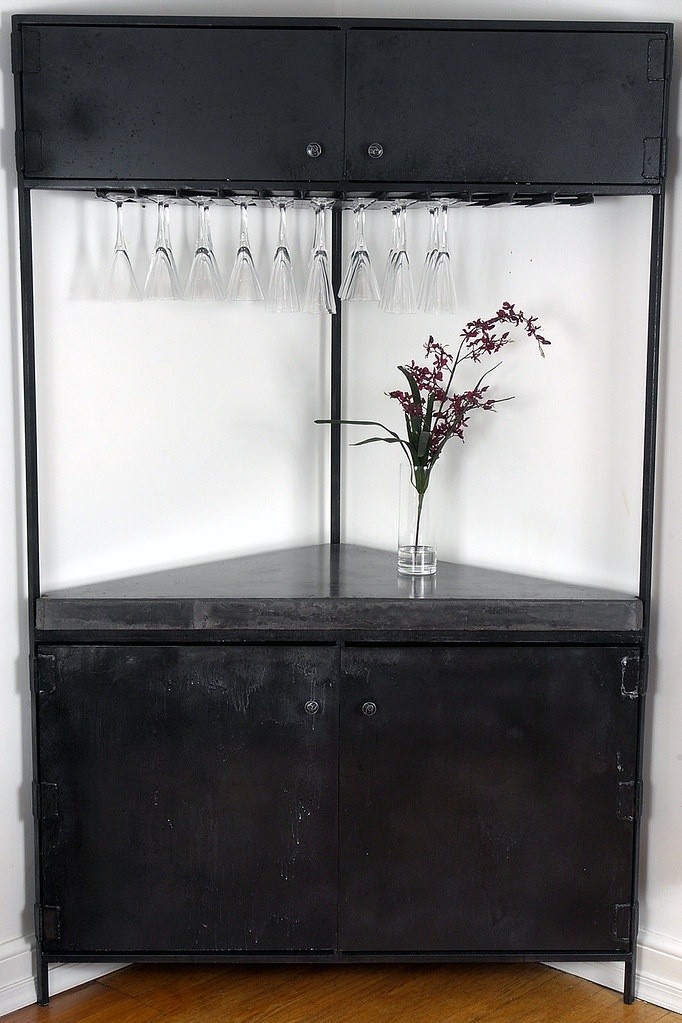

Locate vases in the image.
[397,462,439,576]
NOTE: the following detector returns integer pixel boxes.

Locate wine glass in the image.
[99,192,141,304]
[376,198,420,312]
[333,198,382,301]
[222,196,266,302]
[179,195,226,302]
[262,196,300,311]
[299,197,339,314]
[139,195,185,302]
[420,200,461,313]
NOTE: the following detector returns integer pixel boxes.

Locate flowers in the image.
[313,301,552,565]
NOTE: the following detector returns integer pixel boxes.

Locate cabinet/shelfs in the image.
[10,14,676,1005]
